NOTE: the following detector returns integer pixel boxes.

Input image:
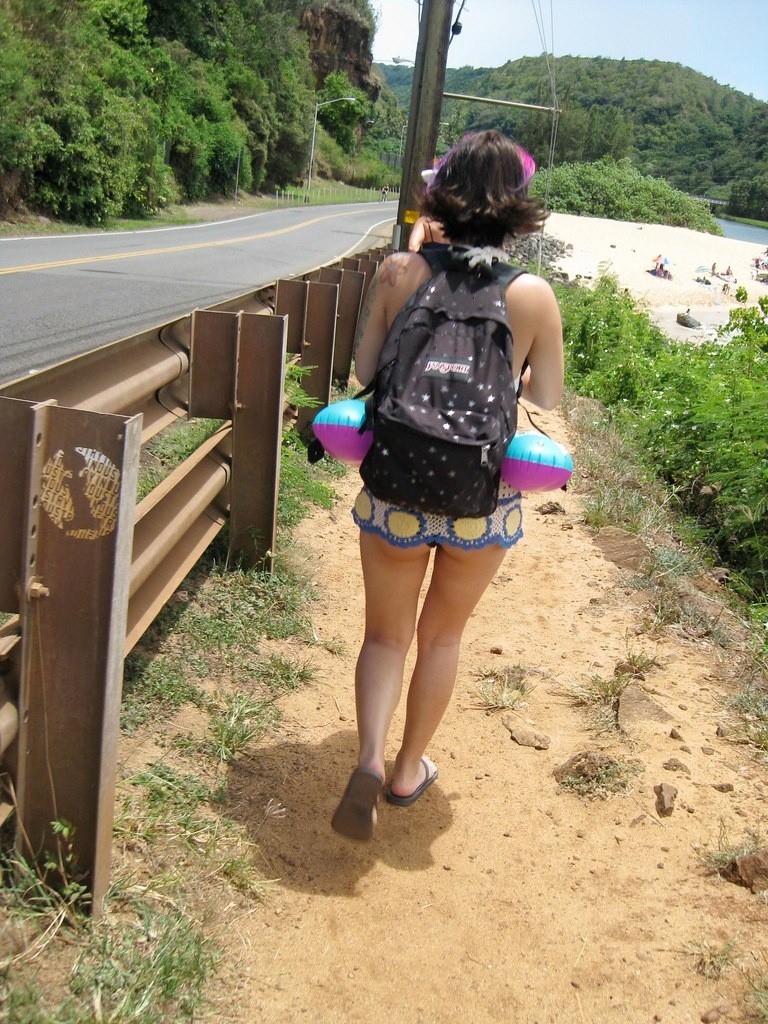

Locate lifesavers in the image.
[311,397,574,493]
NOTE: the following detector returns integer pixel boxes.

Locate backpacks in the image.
[359,249,529,517]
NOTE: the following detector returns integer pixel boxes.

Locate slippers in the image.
[330,766,384,840]
[387,756,439,806]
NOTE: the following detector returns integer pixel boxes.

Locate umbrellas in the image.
[651,256,676,267]
[752,255,765,262]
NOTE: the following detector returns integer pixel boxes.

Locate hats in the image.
[421,169,432,183]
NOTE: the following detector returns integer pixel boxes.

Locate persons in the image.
[332,128,566,841]
[725,266,732,275]
[711,263,717,276]
[657,264,669,278]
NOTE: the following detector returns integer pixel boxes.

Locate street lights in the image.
[399,122,450,166]
[307,97,356,192]
